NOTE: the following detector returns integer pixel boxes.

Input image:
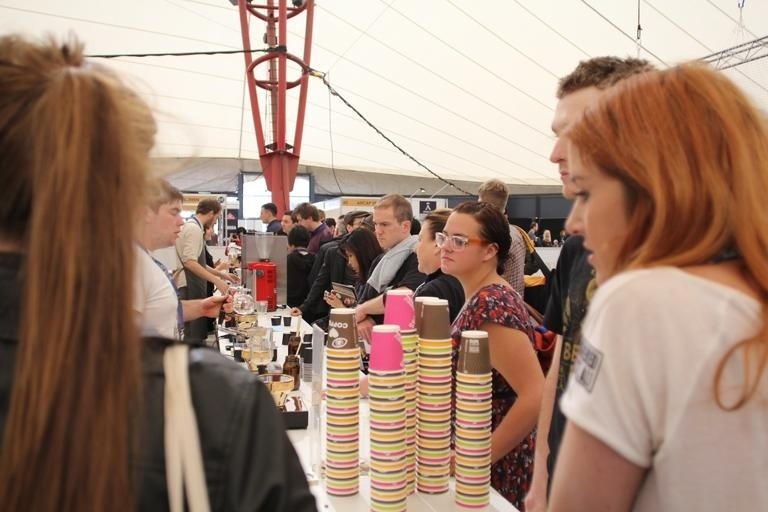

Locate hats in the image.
[343,211,370,225]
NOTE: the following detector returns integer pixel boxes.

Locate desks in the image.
[215,303,518,512]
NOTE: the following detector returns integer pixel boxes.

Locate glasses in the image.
[434,232,485,252]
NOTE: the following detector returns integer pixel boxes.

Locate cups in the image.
[232,267,241,278]
[218,286,313,408]
[326,288,493,512]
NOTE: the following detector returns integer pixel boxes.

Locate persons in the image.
[231,194,466,402]
[519,54,660,512]
[528,220,538,243]
[477,180,532,301]
[546,59,767,512]
[174,193,241,349]
[441,200,546,512]
[0,24,319,512]
[132,178,234,340]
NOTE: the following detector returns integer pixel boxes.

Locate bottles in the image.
[228,245,242,265]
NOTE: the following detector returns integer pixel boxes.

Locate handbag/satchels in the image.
[523,269,557,312]
[529,303,559,370]
